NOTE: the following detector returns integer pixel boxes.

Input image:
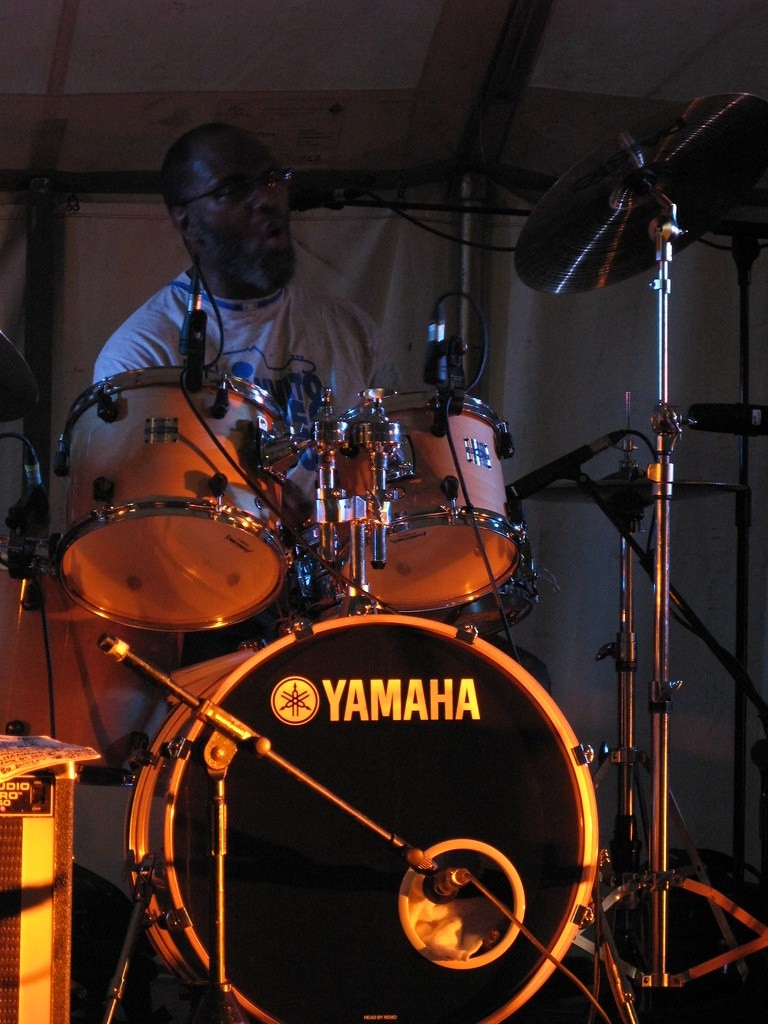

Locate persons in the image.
[92,122,437,747]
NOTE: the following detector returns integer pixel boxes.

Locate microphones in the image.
[425,303,449,384]
[22,448,50,533]
[423,870,472,905]
[293,187,369,212]
[505,431,627,501]
[179,267,207,355]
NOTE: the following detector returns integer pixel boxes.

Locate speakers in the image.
[0,761,74,1024]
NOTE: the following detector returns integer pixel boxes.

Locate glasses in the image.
[179,166,296,211]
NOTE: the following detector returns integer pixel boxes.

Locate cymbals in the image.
[0,330,42,422]
[512,92,768,298]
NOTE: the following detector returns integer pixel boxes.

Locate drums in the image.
[317,386,519,613]
[51,368,295,632]
[124,612,601,1024]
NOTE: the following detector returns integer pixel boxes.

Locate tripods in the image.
[571,220,768,1024]
